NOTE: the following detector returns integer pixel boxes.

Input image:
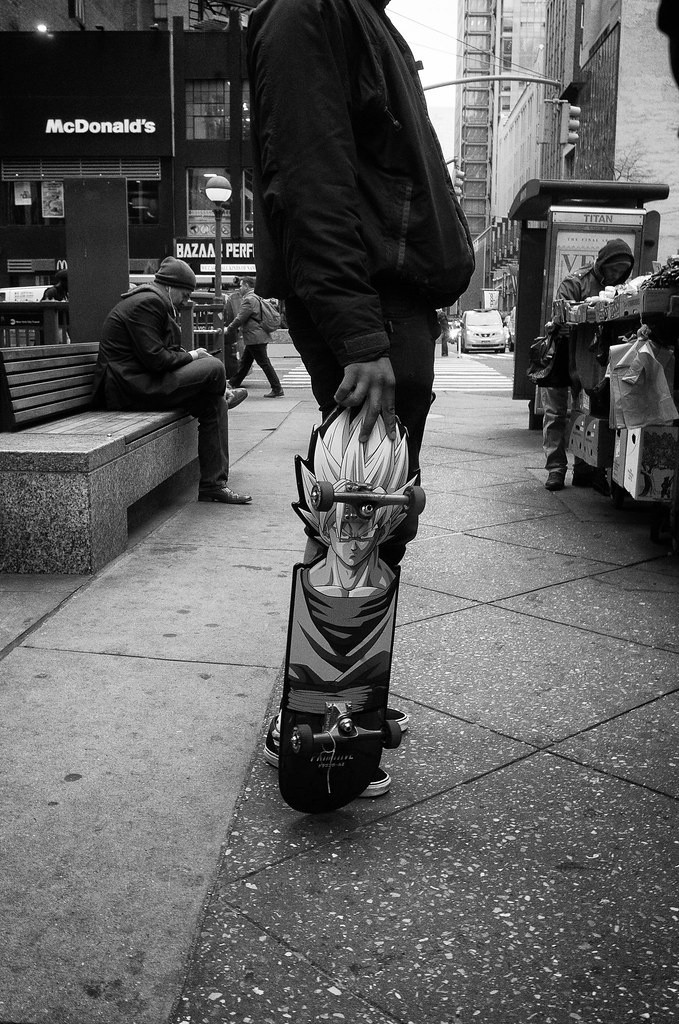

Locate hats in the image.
[602,254,632,268]
[154,256,196,291]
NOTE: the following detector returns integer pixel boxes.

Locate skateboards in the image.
[277,393,426,815]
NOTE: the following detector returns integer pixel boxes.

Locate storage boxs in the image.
[566,409,679,502]
[552,287,679,324]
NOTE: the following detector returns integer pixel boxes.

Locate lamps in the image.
[95,25,105,31]
[149,25,160,31]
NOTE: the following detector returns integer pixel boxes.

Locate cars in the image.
[456,310,507,353]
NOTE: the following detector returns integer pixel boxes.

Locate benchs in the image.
[0,339,199,576]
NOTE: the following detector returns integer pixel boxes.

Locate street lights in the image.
[205,175,233,329]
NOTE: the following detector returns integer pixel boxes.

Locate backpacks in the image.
[249,294,281,332]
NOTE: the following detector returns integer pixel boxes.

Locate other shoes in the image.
[384,704,411,733]
[264,714,392,798]
[264,390,285,398]
[226,380,232,387]
[546,471,565,489]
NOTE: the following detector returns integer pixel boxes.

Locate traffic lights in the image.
[452,168,466,196]
[560,102,581,144]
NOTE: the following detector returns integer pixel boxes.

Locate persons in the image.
[224,276,285,398]
[251,0,477,800]
[539,238,636,490]
[439,313,449,356]
[90,256,252,505]
[40,270,71,345]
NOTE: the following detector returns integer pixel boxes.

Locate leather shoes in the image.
[198,486,253,504]
[226,388,248,409]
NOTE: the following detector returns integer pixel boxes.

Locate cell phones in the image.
[208,349,222,354]
